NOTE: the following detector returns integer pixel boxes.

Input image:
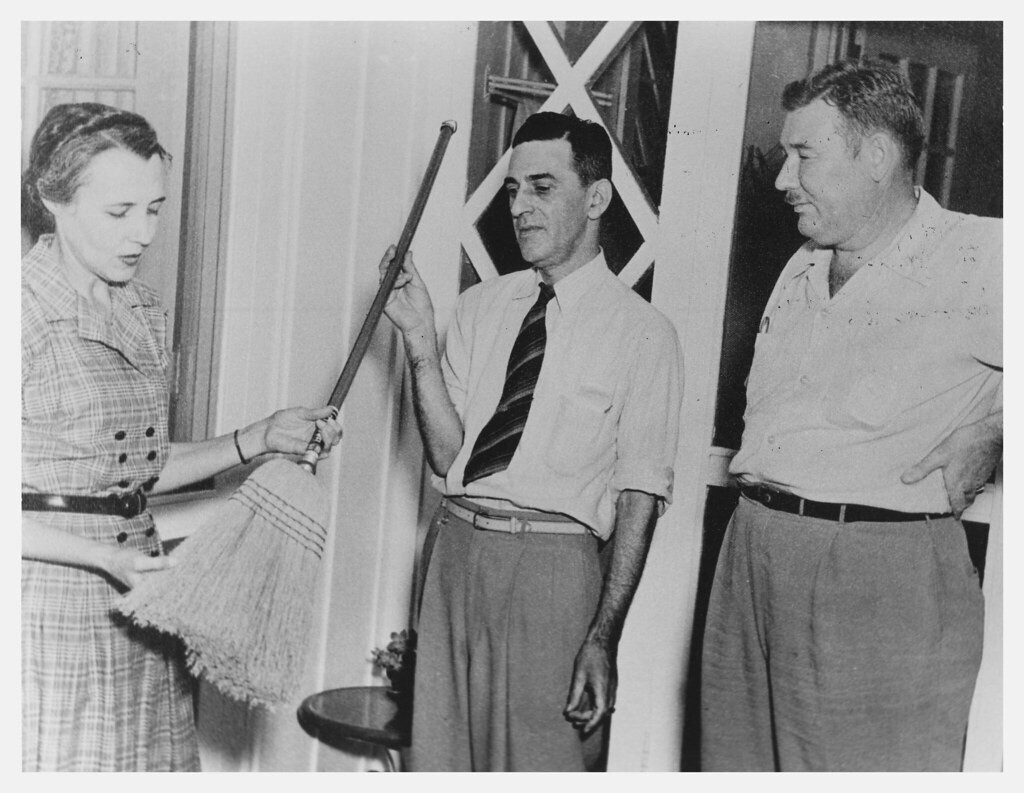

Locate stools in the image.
[299,684,413,770]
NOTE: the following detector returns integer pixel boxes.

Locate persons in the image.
[699,57,1003,772]
[21,102,343,772]
[378,110,684,772]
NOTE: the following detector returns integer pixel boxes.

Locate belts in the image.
[21,482,148,519]
[736,473,954,522]
[440,495,591,535]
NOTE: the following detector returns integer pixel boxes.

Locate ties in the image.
[462,283,556,487]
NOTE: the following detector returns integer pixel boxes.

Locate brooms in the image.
[106,120,460,714]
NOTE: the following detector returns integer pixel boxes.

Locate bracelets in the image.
[234,429,251,465]
